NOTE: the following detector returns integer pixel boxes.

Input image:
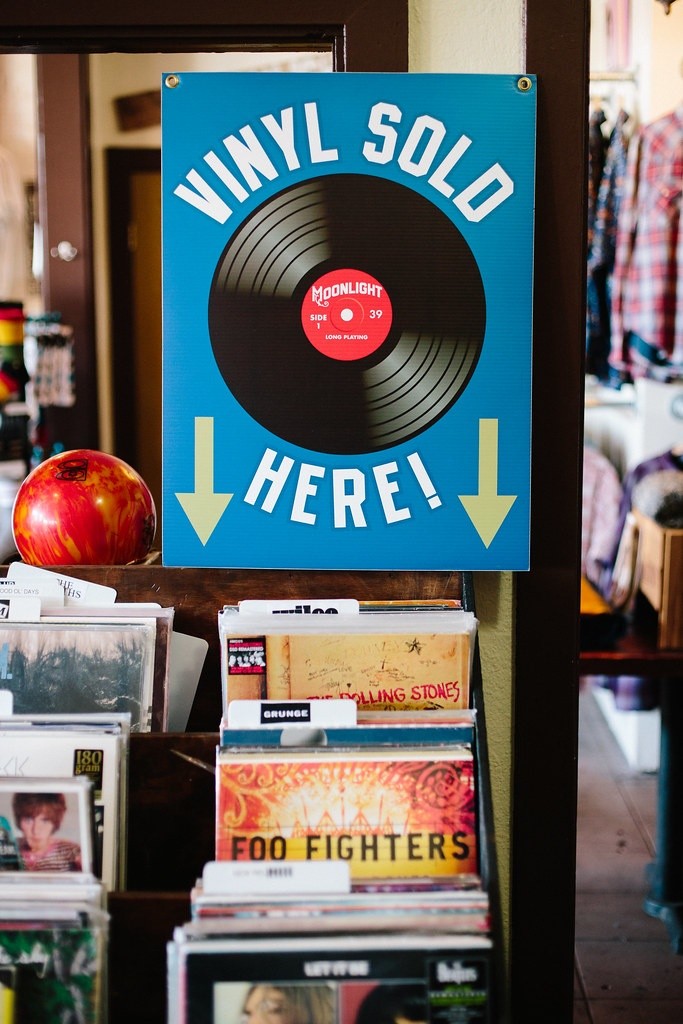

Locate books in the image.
[0,598,497,1023]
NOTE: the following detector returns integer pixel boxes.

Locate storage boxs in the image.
[632,508,683,645]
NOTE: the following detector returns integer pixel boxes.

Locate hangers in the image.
[592,79,639,139]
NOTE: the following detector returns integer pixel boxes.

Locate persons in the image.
[241,985,335,1024]
[13,793,81,873]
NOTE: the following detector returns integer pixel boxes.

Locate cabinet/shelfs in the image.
[0,0,592,1024]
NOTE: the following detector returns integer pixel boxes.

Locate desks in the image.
[576,625,683,956]
